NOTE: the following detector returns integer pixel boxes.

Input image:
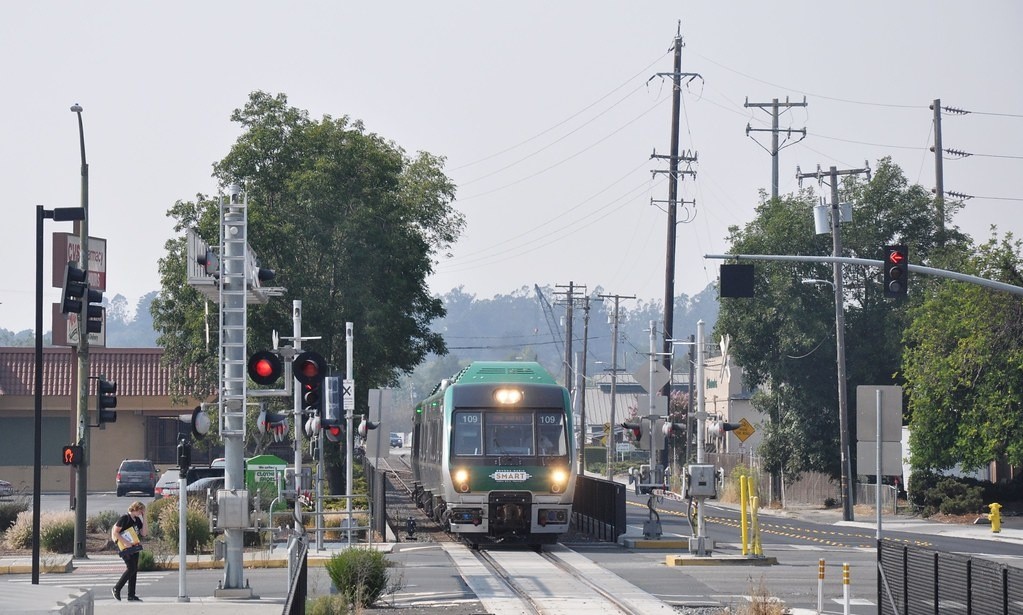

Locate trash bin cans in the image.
[247,456,287,512]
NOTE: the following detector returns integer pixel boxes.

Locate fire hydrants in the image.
[989,503,1005,534]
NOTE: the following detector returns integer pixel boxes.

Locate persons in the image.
[525,427,554,454]
[111,501,147,603]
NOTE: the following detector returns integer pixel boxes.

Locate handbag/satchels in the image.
[111,512,129,544]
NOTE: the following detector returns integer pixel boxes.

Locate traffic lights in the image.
[257,409,286,436]
[200,249,220,277]
[62,445,82,464]
[248,351,282,385]
[358,419,381,441]
[326,425,345,443]
[884,244,910,296]
[59,263,85,312]
[83,285,103,333]
[97,374,117,433]
[179,406,209,441]
[302,383,323,419]
[715,420,741,440]
[621,422,642,441]
[293,351,327,386]
[671,423,687,436]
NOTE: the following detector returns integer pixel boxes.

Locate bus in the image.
[411,359,576,546]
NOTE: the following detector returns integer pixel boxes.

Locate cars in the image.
[388,433,402,449]
[0,480,15,497]
[208,456,248,469]
[186,477,225,513]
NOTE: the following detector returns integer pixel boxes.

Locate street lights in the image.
[802,280,853,522]
[71,104,91,560]
[32,206,85,584]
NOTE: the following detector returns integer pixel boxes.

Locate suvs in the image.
[154,466,226,501]
[115,459,161,498]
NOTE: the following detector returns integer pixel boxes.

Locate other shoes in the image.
[111,586,121,601]
[127,595,140,601]
